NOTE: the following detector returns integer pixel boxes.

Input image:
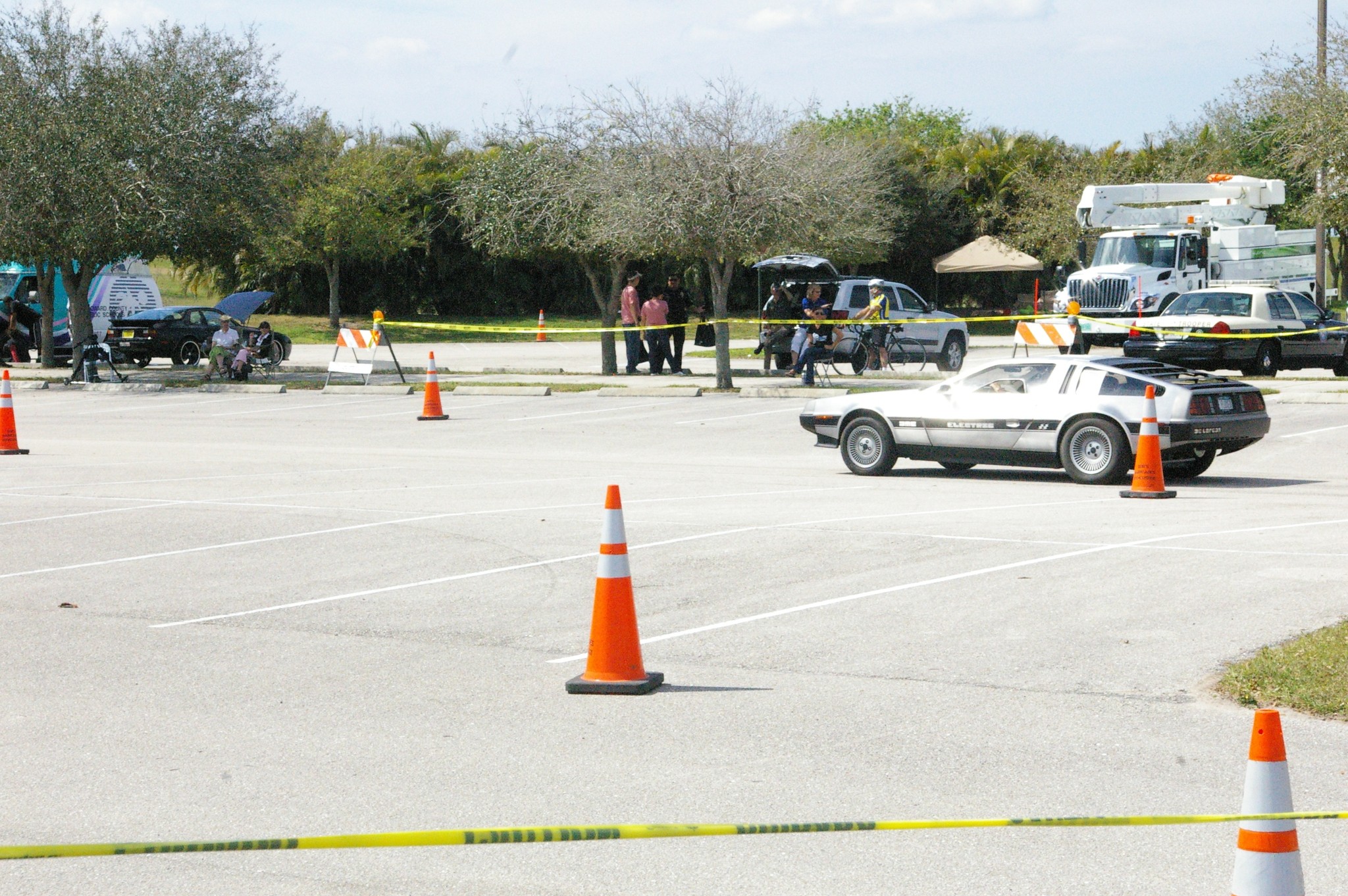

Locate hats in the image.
[258,321,271,329]
[218,314,233,321]
[627,271,643,281]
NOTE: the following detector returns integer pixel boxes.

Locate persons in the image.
[0,296,42,368]
[848,279,890,371]
[200,315,273,384]
[754,281,844,388]
[621,270,703,376]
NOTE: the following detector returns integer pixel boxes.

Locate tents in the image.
[932,235,1044,316]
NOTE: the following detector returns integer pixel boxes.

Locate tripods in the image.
[64,341,125,384]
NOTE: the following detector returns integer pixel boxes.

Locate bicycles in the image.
[831,319,927,377]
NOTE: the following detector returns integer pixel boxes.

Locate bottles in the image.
[775,283,780,293]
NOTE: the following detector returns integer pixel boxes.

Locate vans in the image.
[0,252,165,368]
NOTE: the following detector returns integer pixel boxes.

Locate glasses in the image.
[670,279,678,282]
[815,290,821,294]
[870,287,873,289]
[814,311,825,315]
[222,320,230,323]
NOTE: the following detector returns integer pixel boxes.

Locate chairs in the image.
[83,343,114,382]
[222,326,276,379]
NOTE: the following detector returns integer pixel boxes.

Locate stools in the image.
[813,351,833,387]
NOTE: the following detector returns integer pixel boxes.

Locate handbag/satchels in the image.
[638,340,649,363]
[694,312,716,347]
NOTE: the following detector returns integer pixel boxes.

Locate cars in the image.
[1124,279,1348,377]
[103,306,292,368]
[798,356,1271,485]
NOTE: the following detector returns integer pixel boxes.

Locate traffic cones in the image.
[415,352,449,421]
[0,369,29,455]
[1232,708,1305,896]
[564,482,665,695]
[1119,383,1177,499]
[533,308,549,343]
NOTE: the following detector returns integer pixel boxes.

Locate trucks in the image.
[1052,174,1331,356]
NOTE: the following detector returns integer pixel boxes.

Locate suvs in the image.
[752,252,970,371]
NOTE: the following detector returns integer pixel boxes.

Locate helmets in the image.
[868,279,884,287]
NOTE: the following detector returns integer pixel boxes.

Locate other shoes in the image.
[754,343,765,355]
[201,374,211,381]
[672,371,687,376]
[234,369,243,374]
[626,370,642,375]
[785,371,795,377]
[786,363,794,370]
[650,372,663,376]
[219,367,227,374]
